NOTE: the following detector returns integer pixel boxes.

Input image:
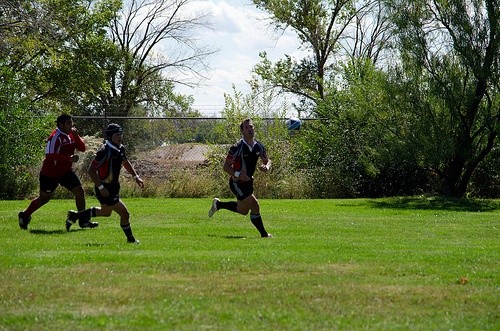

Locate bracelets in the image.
[133,175,138,179]
[98,185,104,190]
[235,171,240,177]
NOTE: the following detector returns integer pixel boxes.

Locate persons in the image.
[209,119,272,237]
[18,113,98,229]
[66,124,144,245]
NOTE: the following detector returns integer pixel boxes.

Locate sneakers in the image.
[208,197,221,218]
[261,234,273,238]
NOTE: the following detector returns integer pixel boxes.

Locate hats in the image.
[106,123,123,142]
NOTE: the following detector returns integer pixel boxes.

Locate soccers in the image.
[284,116,303,132]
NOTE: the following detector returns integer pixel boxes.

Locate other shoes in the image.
[127,240,139,244]
[66,210,77,232]
[80,221,98,228]
[19,211,31,230]
